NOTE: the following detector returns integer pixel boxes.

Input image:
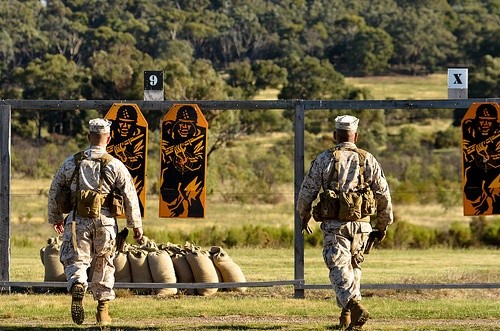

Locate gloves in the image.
[300,214,313,235]
[370,223,387,244]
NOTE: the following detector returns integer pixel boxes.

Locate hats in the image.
[88,118,112,133]
[334,114,360,130]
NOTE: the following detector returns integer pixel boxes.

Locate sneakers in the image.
[70,283,86,326]
[95,300,111,325]
[338,309,351,331]
[343,303,371,331]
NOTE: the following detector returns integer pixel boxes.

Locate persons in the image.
[296,115,393,331]
[47,118,143,325]
